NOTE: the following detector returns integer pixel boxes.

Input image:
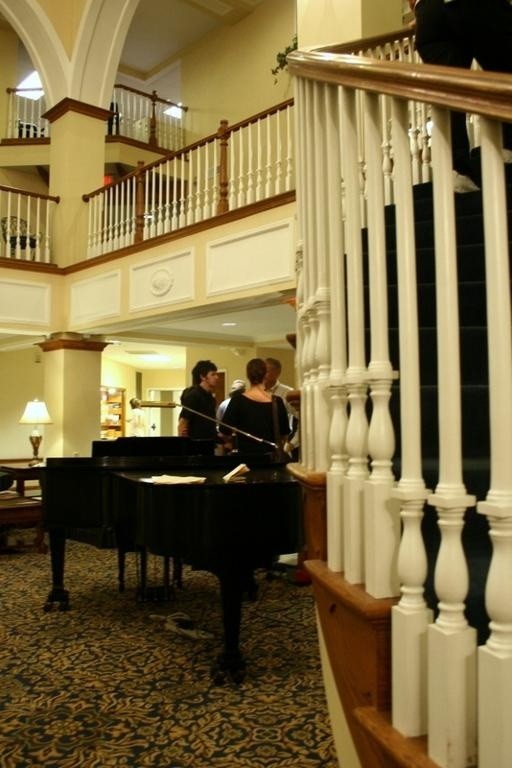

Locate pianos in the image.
[40,437,304,672]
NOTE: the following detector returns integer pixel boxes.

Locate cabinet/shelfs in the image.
[100,384,128,441]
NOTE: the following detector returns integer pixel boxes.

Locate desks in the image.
[0,458,48,497]
[0,491,48,555]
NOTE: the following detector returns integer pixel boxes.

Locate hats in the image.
[229,379,246,395]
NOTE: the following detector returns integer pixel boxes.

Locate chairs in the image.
[1,215,44,262]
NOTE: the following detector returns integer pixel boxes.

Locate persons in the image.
[219,357,290,464]
[216,380,246,439]
[404,0,510,197]
[126,397,149,437]
[263,356,300,460]
[177,360,221,435]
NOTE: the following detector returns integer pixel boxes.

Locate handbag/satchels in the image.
[272,448,291,466]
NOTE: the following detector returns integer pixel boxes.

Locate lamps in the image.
[18,398,53,466]
